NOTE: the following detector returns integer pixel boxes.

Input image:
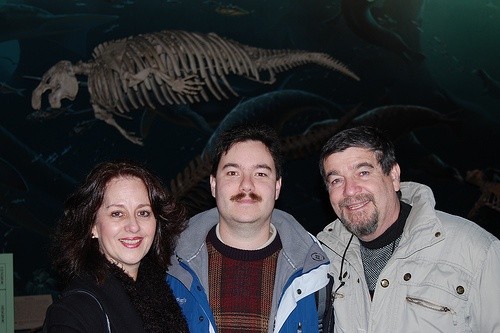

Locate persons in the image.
[315,126,500,333]
[41,161,190,333]
[165,130,330,333]
